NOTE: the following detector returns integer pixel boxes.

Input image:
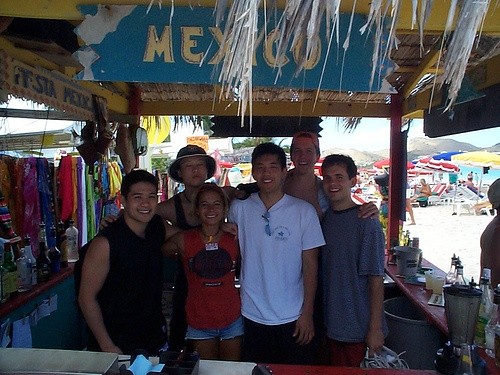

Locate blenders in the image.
[434,277,483,375]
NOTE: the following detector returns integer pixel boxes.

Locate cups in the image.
[433,276,444,294]
[424,271,433,290]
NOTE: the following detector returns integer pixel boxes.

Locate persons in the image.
[480,178,500,304]
[78,171,237,365]
[169,170,479,226]
[99,145,245,231]
[236,132,379,218]
[314,154,388,368]
[228,143,325,365]
[161,183,244,362]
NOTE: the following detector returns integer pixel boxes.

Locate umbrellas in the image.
[210,147,500,176]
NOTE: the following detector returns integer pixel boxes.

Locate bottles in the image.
[484,288,500,358]
[446,254,461,284]
[36,224,52,283]
[65,220,79,262]
[16,250,31,292]
[24,237,37,285]
[494,321,500,367]
[412,238,419,247]
[474,276,493,348]
[482,268,494,302]
[2,243,18,293]
[57,222,68,268]
[0,266,10,304]
[452,266,466,285]
[48,227,61,272]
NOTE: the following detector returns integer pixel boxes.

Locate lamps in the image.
[436,73,487,110]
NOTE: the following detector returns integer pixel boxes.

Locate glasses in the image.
[262,210,271,236]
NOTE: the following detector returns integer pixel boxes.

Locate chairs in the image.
[405,181,497,219]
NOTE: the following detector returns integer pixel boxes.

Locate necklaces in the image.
[198,227,221,236]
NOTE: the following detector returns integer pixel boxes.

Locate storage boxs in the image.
[130,348,200,375]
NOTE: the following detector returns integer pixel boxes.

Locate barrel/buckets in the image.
[383,296,441,370]
[394,246,421,276]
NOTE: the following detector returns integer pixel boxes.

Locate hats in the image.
[488,178,500,208]
[168,145,216,183]
[293,131,320,147]
